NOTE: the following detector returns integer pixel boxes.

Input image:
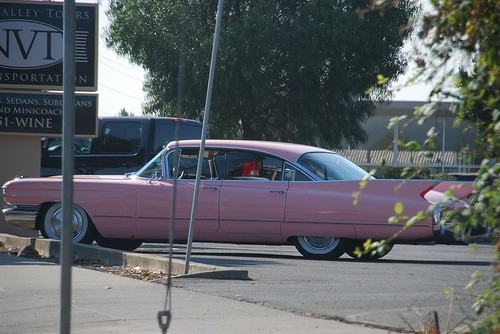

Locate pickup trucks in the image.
[39,116,211,178]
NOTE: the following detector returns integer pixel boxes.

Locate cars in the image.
[1,140,476,260]
